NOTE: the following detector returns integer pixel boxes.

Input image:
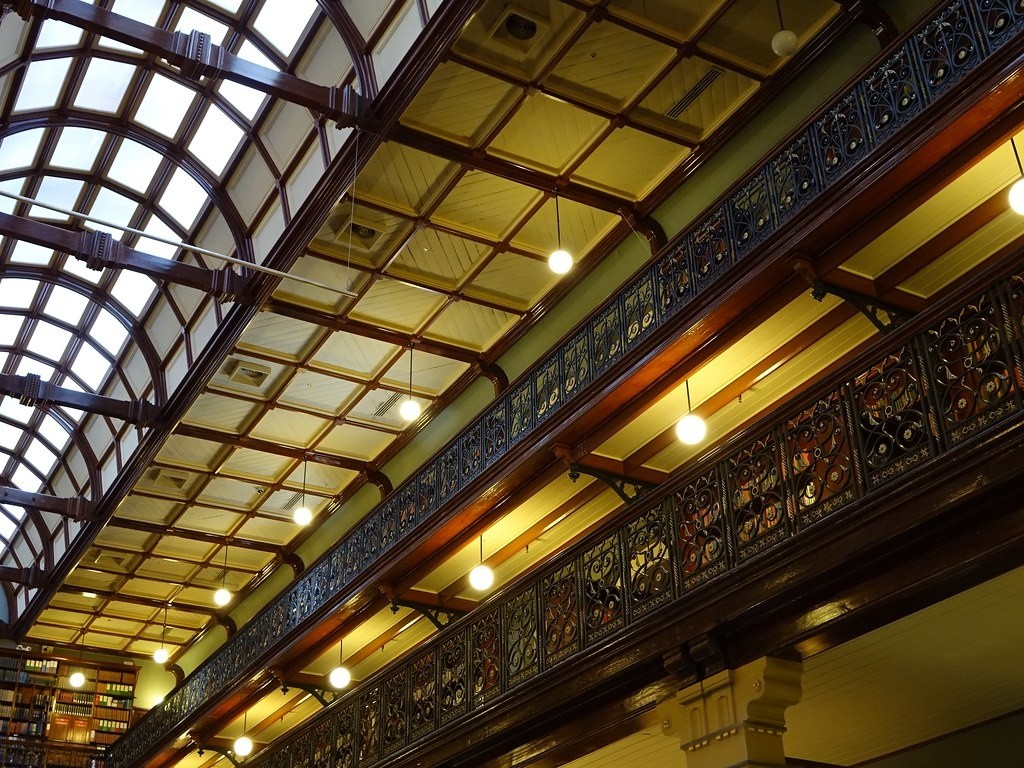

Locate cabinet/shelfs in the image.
[0,647,141,768]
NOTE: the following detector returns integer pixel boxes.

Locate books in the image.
[0,656,135,744]
